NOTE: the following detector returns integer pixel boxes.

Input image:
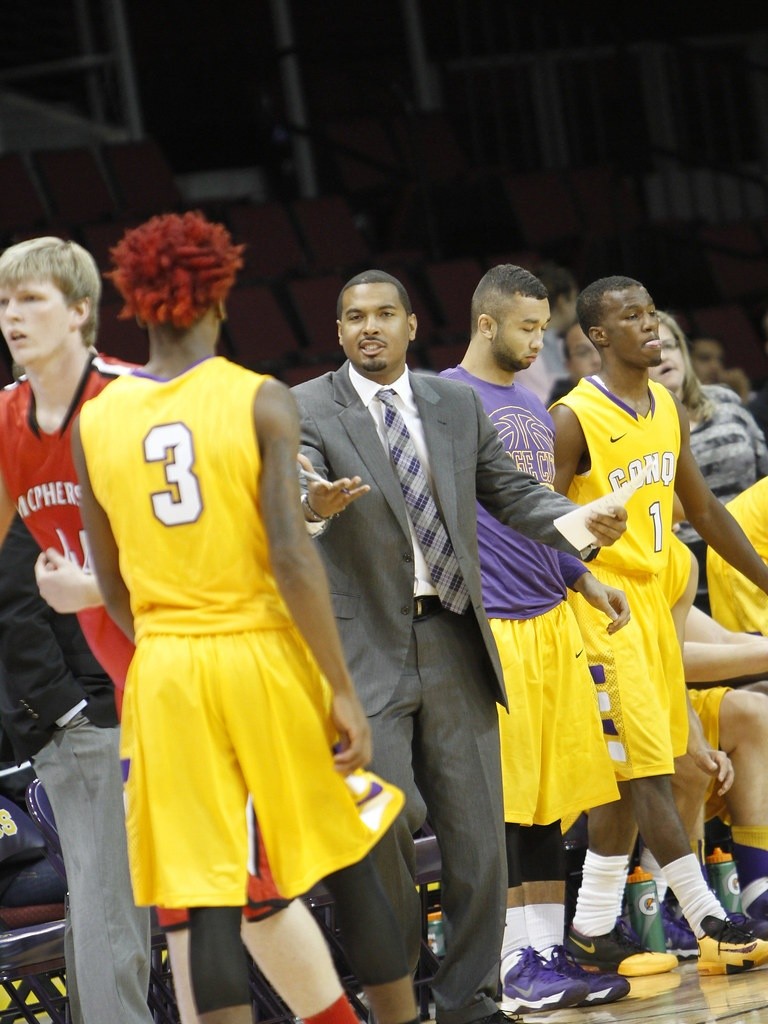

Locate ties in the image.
[376,386,471,615]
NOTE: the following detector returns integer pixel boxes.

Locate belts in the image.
[412,594,445,619]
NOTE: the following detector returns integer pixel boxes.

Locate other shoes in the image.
[472,1010,523,1024]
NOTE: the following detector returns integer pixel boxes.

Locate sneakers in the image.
[619,901,700,957]
[729,913,768,941]
[542,945,631,1006]
[566,917,679,976]
[501,945,590,1015]
[695,911,768,975]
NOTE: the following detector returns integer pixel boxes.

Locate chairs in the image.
[0,116,768,387]
[0,774,441,1024]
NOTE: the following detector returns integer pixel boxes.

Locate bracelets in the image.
[303,493,333,523]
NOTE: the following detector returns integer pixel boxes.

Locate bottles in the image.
[415,906,446,960]
[625,865,667,953]
[705,846,742,915]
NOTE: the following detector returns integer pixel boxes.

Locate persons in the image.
[0,212,768,1024]
